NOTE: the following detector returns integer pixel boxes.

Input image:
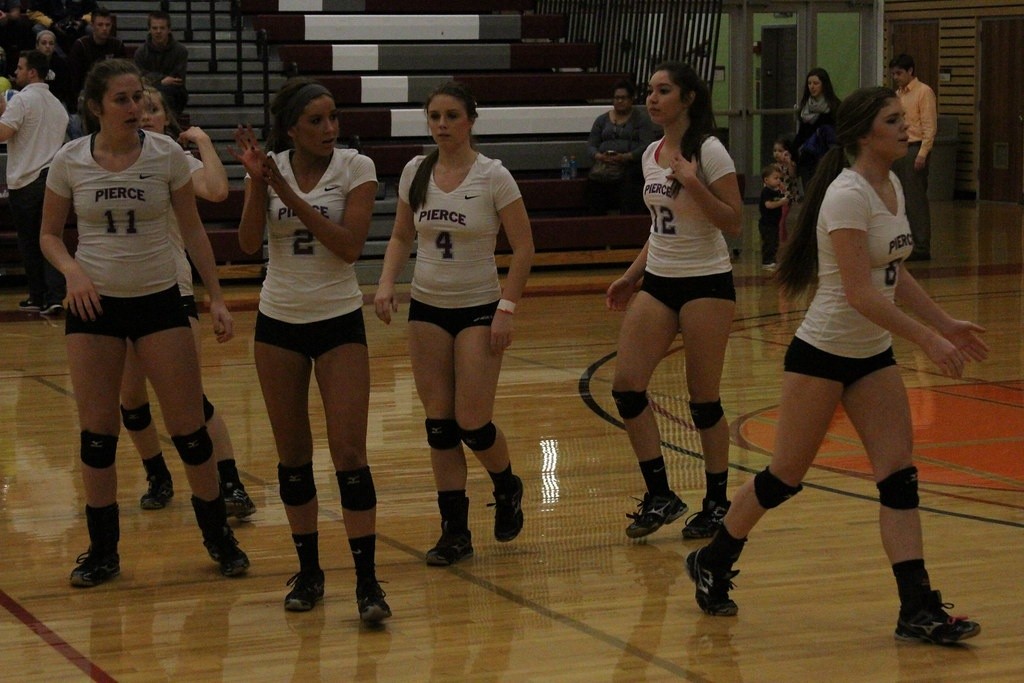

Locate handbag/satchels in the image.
[587,159,629,181]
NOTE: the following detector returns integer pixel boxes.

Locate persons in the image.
[889,55,937,261]
[226,78,393,625]
[40,57,255,586]
[687,86,990,642]
[570,81,653,215]
[373,84,535,567]
[606,61,744,537]
[757,68,843,271]
[0,0,187,308]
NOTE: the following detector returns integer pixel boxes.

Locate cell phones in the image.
[606,150,616,156]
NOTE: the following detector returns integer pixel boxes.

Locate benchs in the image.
[0,0,650,267]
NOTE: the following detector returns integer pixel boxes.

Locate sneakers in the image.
[356,583,392,622]
[202,523,250,576]
[19,297,40,310]
[895,590,981,645]
[686,544,740,616]
[285,568,325,611]
[219,479,257,519]
[487,475,523,542]
[39,304,63,316]
[626,489,688,538]
[682,498,731,538]
[141,473,174,509]
[426,520,473,566]
[70,544,120,587]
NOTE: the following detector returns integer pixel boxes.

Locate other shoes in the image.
[903,250,932,263]
[763,260,777,269]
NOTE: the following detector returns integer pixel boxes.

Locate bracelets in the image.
[497,299,515,314]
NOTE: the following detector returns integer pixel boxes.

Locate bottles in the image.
[561,155,577,180]
